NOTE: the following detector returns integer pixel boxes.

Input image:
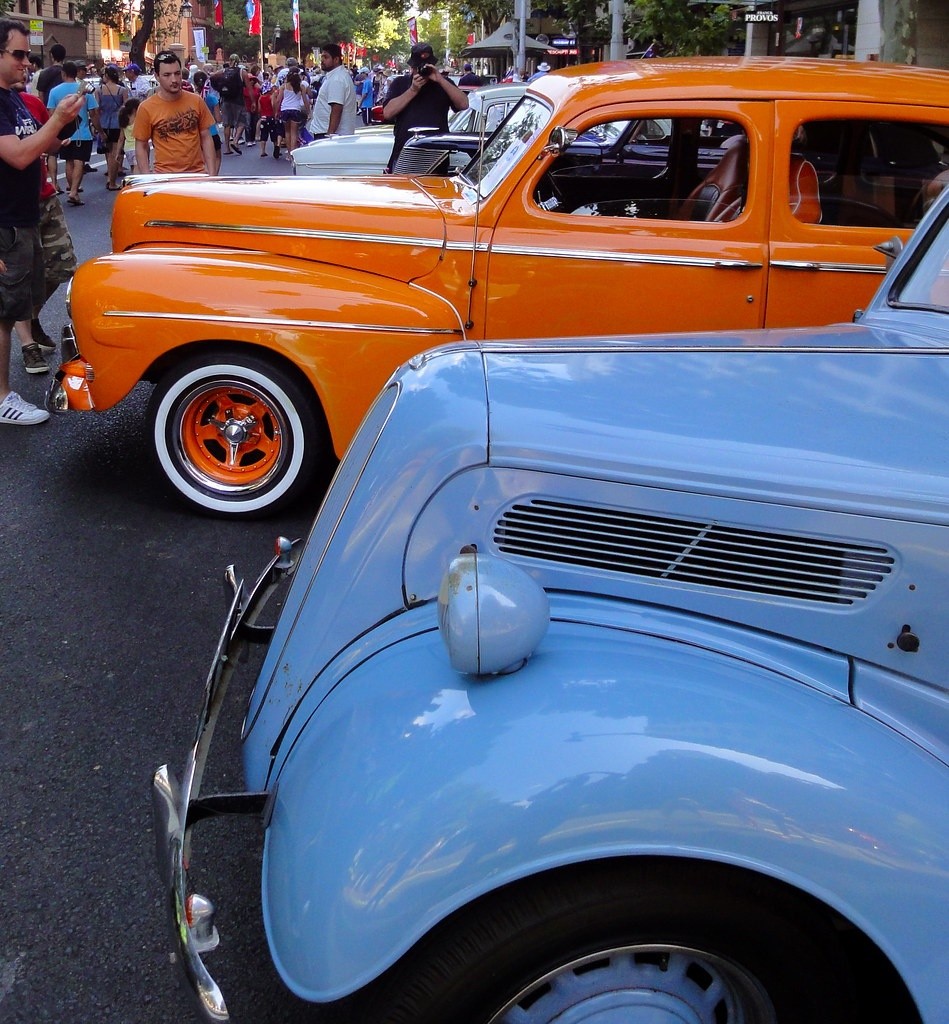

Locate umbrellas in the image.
[457,22,555,75]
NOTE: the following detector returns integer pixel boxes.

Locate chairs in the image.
[664,140,823,224]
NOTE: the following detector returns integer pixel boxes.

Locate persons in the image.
[0,19,86,424]
[9,68,76,373]
[26,44,550,204]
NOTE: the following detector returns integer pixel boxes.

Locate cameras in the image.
[76,79,95,97]
[417,62,433,78]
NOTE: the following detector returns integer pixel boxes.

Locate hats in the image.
[536,61,550,72]
[73,54,304,84]
[407,43,437,71]
[356,66,370,75]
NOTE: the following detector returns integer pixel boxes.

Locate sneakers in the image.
[30,324,57,351]
[0,392,49,424]
[21,343,49,371]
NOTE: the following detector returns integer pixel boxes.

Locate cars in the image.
[44,57,949,526]
[287,81,671,175]
[151,181,949,1024]
[370,85,478,127]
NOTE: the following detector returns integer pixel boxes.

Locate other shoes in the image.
[47,132,306,206]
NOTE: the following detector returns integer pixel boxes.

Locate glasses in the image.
[156,53,175,62]
[0,48,30,61]
